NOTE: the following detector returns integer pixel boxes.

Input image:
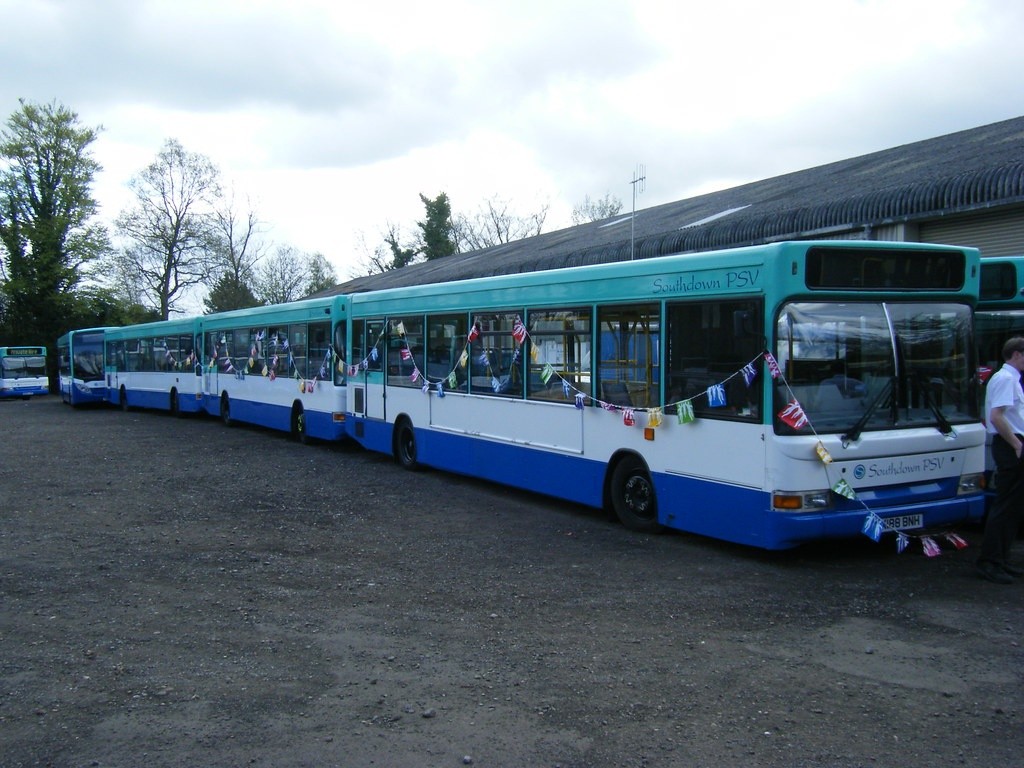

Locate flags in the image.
[395,319,479,398]
[281,339,331,394]
[762,349,967,558]
[511,316,759,430]
[333,328,385,377]
[163,337,193,368]
[475,315,519,393]
[194,336,220,375]
[259,330,278,381]
[221,332,259,380]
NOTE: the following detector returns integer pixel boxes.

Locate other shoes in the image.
[1002,564,1024,577]
[976,561,1014,583]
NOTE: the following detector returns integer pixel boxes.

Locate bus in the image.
[599,257,1024,511]
[202,295,347,446]
[58,327,119,406]
[346,241,980,550]
[0,346,48,399]
[103,314,203,417]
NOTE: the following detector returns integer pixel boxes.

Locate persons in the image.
[968,337,1024,584]
[812,360,867,410]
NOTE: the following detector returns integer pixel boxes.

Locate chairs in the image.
[601,383,633,407]
